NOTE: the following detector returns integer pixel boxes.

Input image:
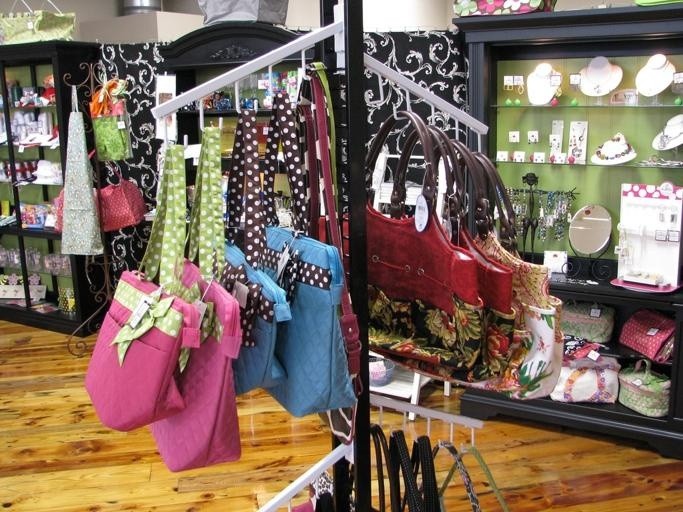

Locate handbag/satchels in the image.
[453,0,558,15]
[341,110,565,401]
[85,62,362,473]
[0,1,76,47]
[197,0,290,27]
[548,298,673,419]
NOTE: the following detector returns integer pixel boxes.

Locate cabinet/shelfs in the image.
[155,19,321,267]
[452,2,682,463]
[1,39,158,339]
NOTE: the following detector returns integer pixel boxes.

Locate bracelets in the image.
[496,187,572,243]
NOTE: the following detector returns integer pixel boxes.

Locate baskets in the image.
[369,357,396,386]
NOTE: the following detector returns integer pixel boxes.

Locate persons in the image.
[521,63,562,106]
[633,54,676,98]
[588,133,637,166]
[577,56,624,98]
[649,113,682,151]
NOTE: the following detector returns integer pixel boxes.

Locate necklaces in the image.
[657,129,683,149]
[587,71,610,97]
[595,142,631,159]
